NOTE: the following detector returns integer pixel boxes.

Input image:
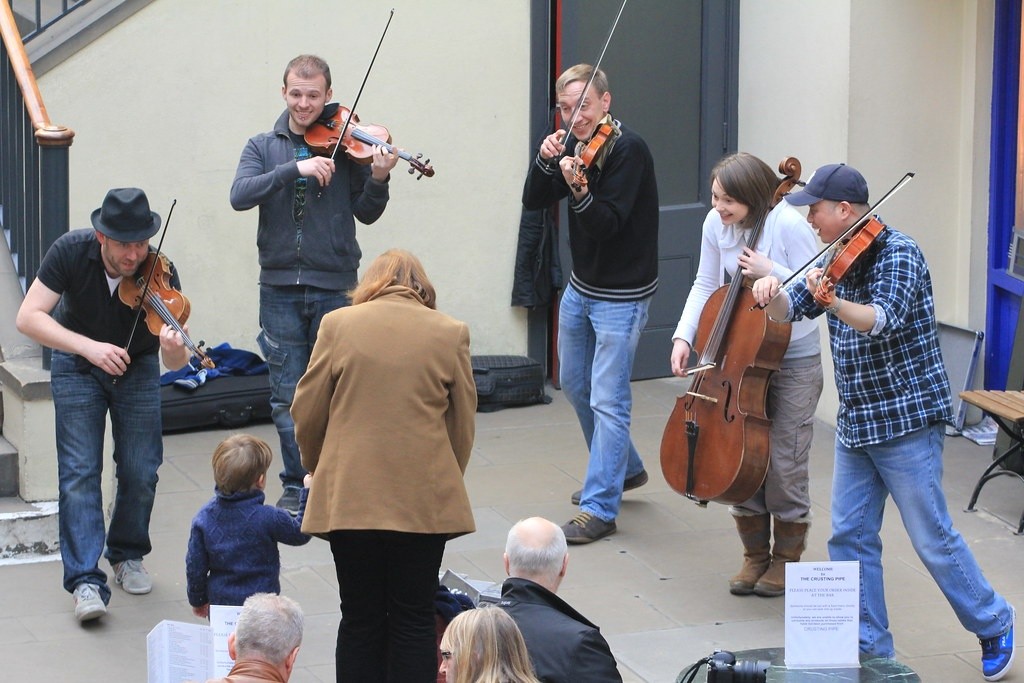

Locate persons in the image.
[439,606,538,683]
[522,65,658,543]
[669,153,821,595]
[185,593,303,683]
[484,517,624,683]
[291,249,476,683]
[753,161,1016,681]
[186,434,312,623]
[231,54,397,526]
[15,188,190,621]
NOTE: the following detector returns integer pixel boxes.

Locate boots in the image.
[728,507,773,596]
[753,510,814,598]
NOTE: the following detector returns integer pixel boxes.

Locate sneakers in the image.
[275,486,301,515]
[561,509,617,542]
[571,468,648,505]
[977,607,1016,681]
[72,582,107,622]
[111,558,154,596]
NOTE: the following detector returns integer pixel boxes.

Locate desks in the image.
[668,641,926,683]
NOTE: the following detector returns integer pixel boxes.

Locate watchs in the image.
[825,296,841,313]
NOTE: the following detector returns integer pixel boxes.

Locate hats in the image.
[783,162,869,207]
[90,188,163,244]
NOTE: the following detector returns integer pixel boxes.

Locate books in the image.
[146,605,243,683]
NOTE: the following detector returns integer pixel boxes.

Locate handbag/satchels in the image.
[471,355,552,413]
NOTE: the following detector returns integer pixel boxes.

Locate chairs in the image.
[960,377,1024,550]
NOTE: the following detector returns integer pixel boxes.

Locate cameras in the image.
[707,650,771,683]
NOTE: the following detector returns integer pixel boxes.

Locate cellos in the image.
[659,154,808,509]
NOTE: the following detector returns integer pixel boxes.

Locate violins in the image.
[811,217,887,307]
[571,120,615,193]
[117,251,215,372]
[305,106,436,181]
